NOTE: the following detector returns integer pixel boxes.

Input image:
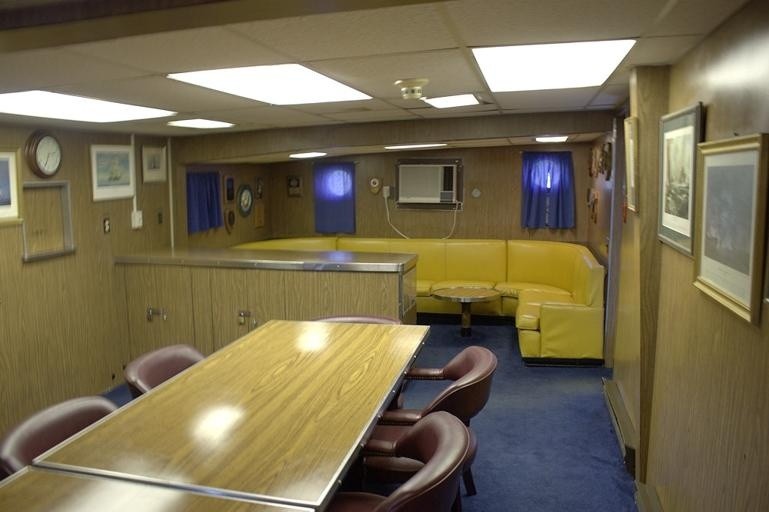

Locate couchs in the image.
[232,237,606,359]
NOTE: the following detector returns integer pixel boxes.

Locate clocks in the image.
[367,176,382,195]
[26,132,64,177]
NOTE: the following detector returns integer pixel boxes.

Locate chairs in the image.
[378,344,498,497]
[0,396,118,471]
[123,343,204,399]
[323,412,478,512]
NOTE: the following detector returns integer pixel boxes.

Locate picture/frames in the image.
[90,144,135,202]
[657,101,701,258]
[691,134,768,327]
[287,175,305,197]
[623,117,638,213]
[0,152,19,220]
[19,180,76,264]
[223,173,265,234]
[587,141,613,224]
[141,143,168,183]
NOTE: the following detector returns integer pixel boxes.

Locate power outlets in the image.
[382,185,390,198]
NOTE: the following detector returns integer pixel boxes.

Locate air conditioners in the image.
[397,163,458,204]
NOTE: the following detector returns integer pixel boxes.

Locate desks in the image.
[31,319,432,512]
[0,465,319,512]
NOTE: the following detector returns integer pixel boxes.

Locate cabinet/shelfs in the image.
[116,247,418,364]
